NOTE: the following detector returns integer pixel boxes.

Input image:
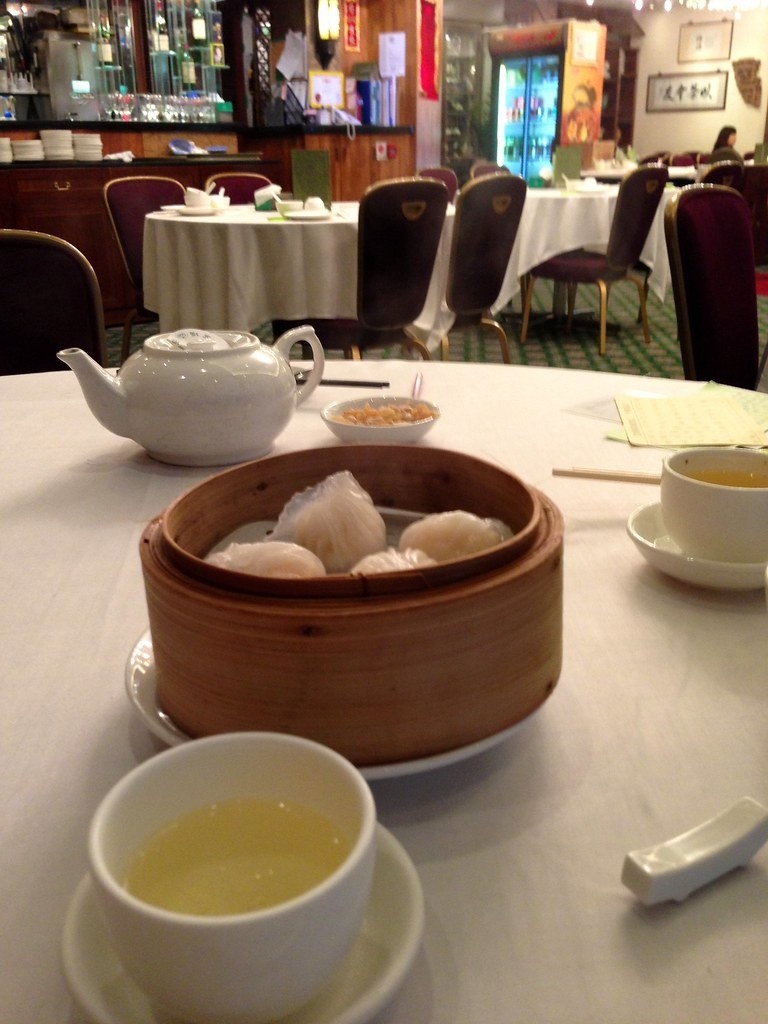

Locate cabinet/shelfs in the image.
[559,4,645,158]
[85,0,229,99]
[0,158,284,326]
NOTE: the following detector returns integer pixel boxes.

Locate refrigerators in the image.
[488,21,608,184]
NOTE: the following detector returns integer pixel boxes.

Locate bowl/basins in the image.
[276,201,302,219]
[305,195,324,213]
[184,185,231,208]
[320,397,440,447]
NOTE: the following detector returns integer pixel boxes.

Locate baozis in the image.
[200,467,518,576]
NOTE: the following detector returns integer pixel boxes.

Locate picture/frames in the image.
[678,19,734,62]
[645,71,728,112]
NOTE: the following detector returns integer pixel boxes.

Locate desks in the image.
[142,201,454,361]
[0,361,768,1024]
[490,186,681,323]
[581,166,697,186]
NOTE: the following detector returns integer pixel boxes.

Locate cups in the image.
[89,732,381,1023]
[659,448,768,562]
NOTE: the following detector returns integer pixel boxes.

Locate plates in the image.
[124,625,533,784]
[61,819,423,1024]
[289,213,333,220]
[624,504,768,594]
[0,130,102,165]
[160,204,217,214]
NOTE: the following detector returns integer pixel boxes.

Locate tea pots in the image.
[54,324,327,469]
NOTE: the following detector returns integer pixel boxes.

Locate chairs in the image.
[0,154,768,394]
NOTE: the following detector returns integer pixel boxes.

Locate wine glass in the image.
[100,95,217,123]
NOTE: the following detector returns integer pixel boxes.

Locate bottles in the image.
[93,14,113,67]
[151,1,173,53]
[191,1,209,46]
[182,45,198,90]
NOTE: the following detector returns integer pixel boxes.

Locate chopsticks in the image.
[297,378,390,387]
[551,466,662,487]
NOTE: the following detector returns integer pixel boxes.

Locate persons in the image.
[714,126,737,150]
